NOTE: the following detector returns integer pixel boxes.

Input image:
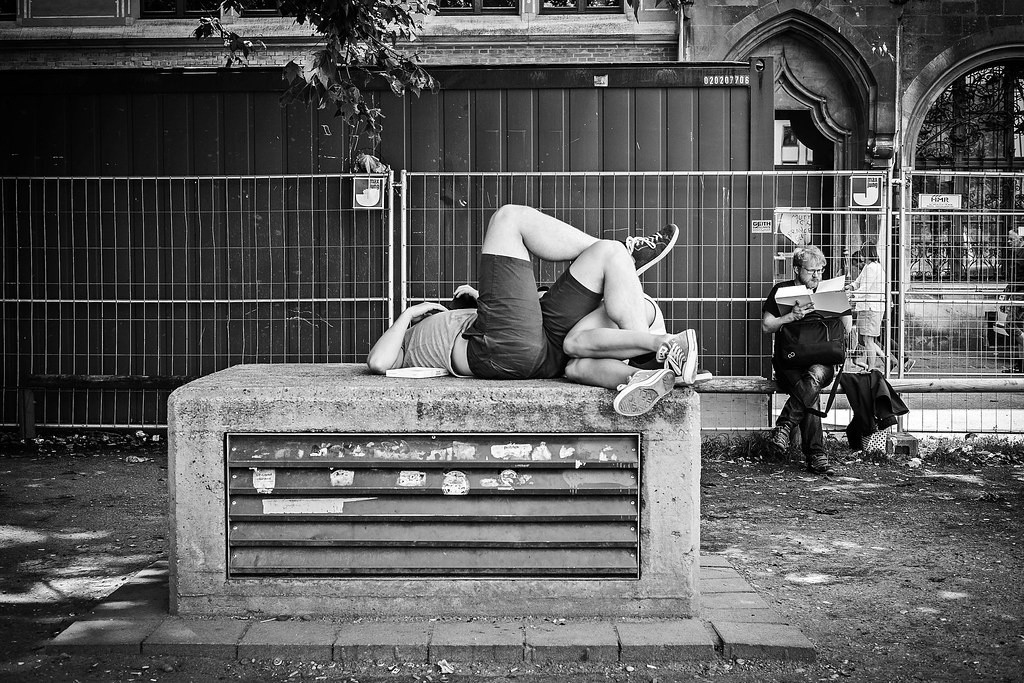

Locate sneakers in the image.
[627,357,713,386]
[807,454,835,475]
[613,369,674,417]
[770,424,791,452]
[623,223,680,277]
[662,328,698,385]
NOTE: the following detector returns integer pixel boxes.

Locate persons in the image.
[850,250,917,375]
[843,244,887,374]
[762,246,854,475]
[446,284,697,417]
[366,204,682,381]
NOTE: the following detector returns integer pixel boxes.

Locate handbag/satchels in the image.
[780,312,845,365]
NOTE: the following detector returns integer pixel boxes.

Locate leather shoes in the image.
[1002,365,1023,373]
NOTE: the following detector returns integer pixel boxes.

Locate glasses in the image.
[798,265,824,274]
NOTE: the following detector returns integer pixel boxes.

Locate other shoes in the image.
[904,360,915,372]
[890,360,897,371]
